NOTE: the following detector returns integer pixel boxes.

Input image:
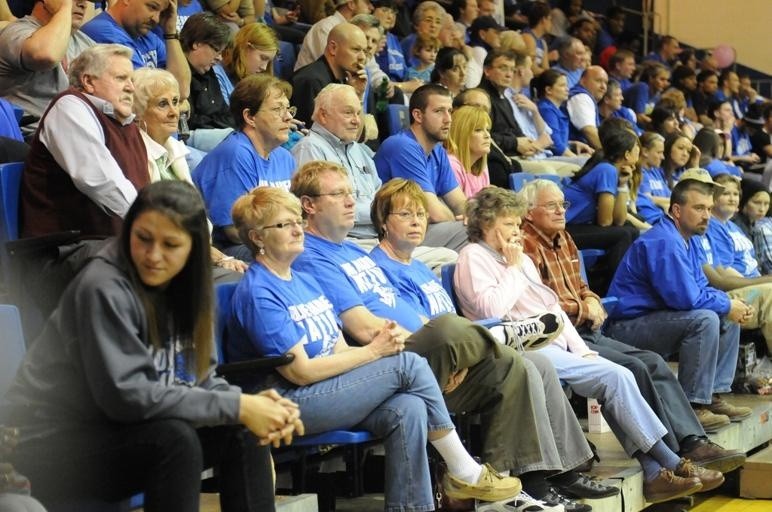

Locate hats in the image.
[738,177,770,212]
[677,168,726,202]
[468,14,509,33]
[741,101,764,129]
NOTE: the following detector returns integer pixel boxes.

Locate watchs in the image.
[360,99,364,105]
[163,30,180,41]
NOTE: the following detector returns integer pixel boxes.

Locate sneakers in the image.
[691,404,730,432]
[441,465,523,502]
[474,487,567,512]
[703,394,753,423]
[485,311,565,351]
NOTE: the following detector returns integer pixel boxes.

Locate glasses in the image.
[304,190,360,202]
[385,210,428,222]
[488,64,517,76]
[254,106,298,119]
[256,219,308,232]
[531,201,570,212]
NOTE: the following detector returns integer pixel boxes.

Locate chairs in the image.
[212,282,375,511]
[0,304,146,512]
[0,161,82,311]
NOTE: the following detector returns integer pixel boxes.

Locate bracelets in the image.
[616,186,627,193]
[242,18,245,26]
[214,254,234,266]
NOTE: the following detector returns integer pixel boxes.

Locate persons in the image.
[693,128,730,179]
[368,177,620,512]
[294,0,375,72]
[349,14,382,152]
[78,0,234,174]
[293,83,459,281]
[732,103,771,175]
[374,83,469,255]
[290,160,564,512]
[408,37,443,82]
[401,1,446,58]
[515,178,747,473]
[750,102,772,172]
[598,117,654,236]
[285,22,367,129]
[1,0,98,144]
[0,182,305,512]
[441,13,461,47]
[755,218,772,275]
[213,23,308,150]
[677,169,772,360]
[445,105,491,199]
[432,45,466,90]
[408,0,477,83]
[453,184,726,503]
[191,74,296,262]
[255,0,295,79]
[659,132,701,192]
[178,12,237,128]
[176,0,203,38]
[566,11,769,128]
[132,66,249,285]
[18,43,149,318]
[637,131,672,216]
[229,186,523,512]
[707,172,762,278]
[397,84,401,88]
[562,131,639,266]
[377,4,402,50]
[605,179,752,433]
[479,0,567,179]
[223,23,280,83]
[732,178,771,277]
[712,129,743,183]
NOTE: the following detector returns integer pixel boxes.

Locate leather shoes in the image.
[675,438,746,473]
[521,483,592,512]
[642,471,703,503]
[553,473,620,500]
[674,457,725,493]
